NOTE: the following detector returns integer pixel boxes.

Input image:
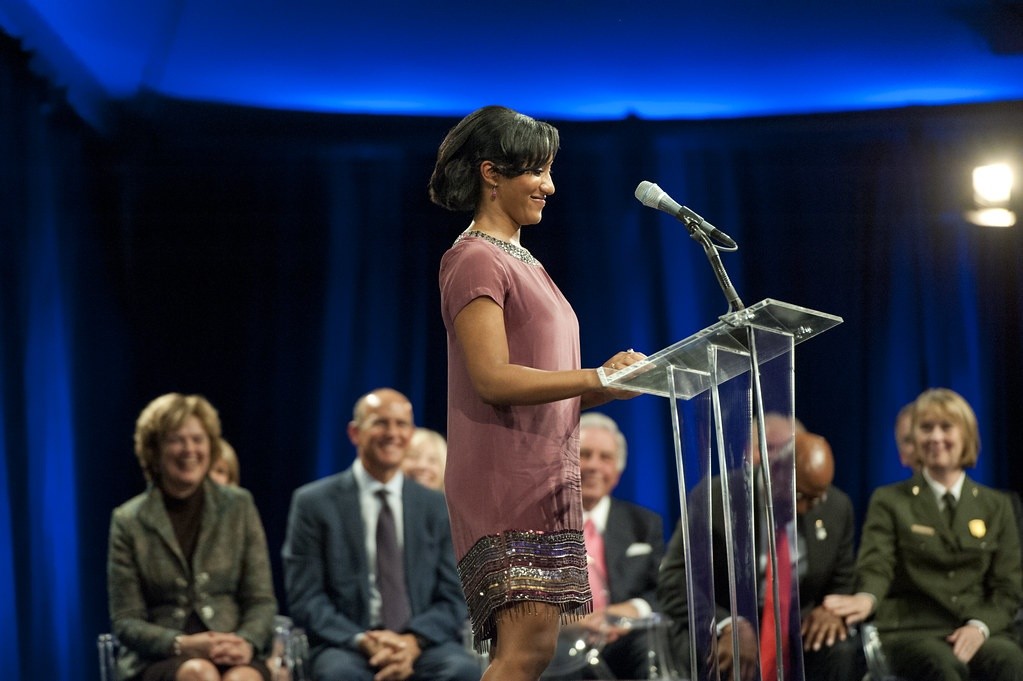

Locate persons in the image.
[108,392,289,681]
[281,387,481,681]
[430,104,659,681]
[823,386,1023,681]
[895,400,925,477]
[538,412,664,681]
[658,413,865,681]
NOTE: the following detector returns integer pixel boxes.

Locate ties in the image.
[760,520,792,680]
[582,519,609,612]
[941,491,957,528]
[374,489,412,633]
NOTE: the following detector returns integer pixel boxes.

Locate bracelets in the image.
[174,636,183,655]
[968,623,987,642]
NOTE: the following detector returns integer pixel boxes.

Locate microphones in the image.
[634,181,737,247]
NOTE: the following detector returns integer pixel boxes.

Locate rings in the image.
[612,364,615,369]
[627,349,635,356]
